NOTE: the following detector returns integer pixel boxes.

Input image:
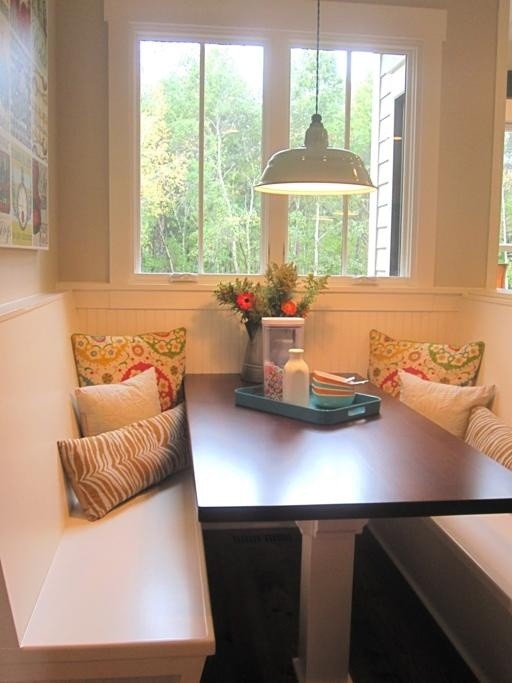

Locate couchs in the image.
[368,293,512,683]
[1,293,218,682]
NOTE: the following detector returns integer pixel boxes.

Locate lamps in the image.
[250,0,378,196]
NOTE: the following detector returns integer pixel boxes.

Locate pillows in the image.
[463,407,512,470]
[74,367,161,438]
[57,402,192,522]
[71,326,186,412]
[395,367,496,441]
[367,329,486,401]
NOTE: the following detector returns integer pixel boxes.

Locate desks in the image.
[183,373,512,683]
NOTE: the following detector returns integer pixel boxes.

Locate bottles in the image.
[282,348,309,407]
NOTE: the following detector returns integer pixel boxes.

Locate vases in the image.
[241,324,263,384]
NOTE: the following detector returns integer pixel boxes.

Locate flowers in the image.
[214,260,332,340]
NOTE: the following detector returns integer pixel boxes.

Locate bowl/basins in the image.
[310,371,356,408]
